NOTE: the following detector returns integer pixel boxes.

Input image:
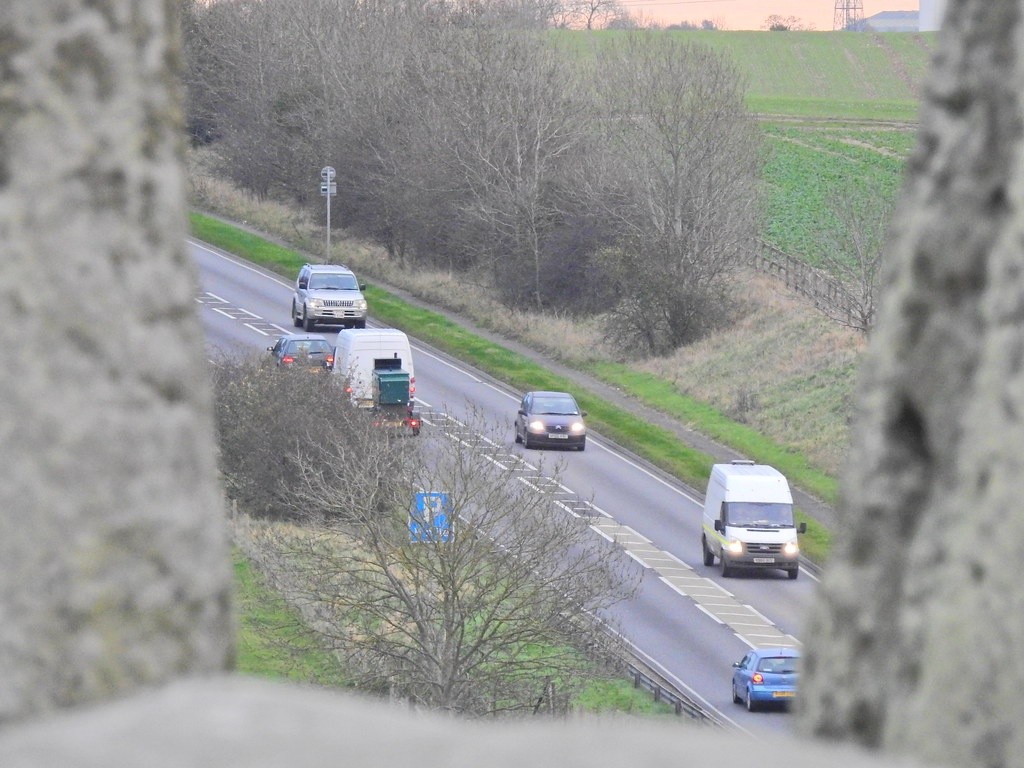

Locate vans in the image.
[701,460,807,580]
[327,329,415,412]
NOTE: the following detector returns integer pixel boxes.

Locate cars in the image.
[514,391,588,451]
[267,334,333,373]
[732,648,803,712]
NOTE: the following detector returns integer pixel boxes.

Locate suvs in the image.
[292,263,368,332]
